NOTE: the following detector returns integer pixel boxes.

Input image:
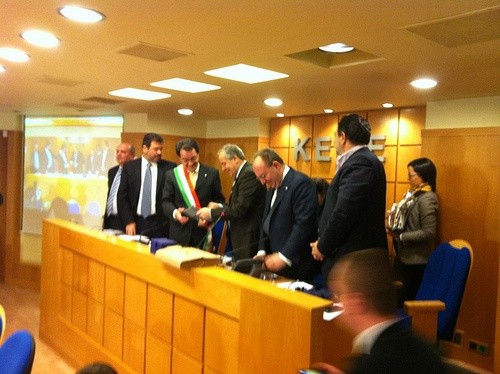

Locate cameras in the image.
[298,368,327,374]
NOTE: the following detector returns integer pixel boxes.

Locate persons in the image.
[26,180,84,226]
[41,141,57,173]
[251,148,319,284]
[161,137,226,248]
[56,144,70,174]
[304,249,446,374]
[102,141,136,234]
[307,113,387,300]
[70,142,119,177]
[117,131,179,240]
[195,143,268,264]
[312,177,330,232]
[386,157,440,315]
[30,143,43,173]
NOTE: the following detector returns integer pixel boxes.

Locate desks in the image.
[38,215,345,374]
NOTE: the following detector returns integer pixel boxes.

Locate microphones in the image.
[222,235,273,261]
[196,210,228,248]
[139,220,169,243]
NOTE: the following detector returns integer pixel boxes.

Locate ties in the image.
[141,162,152,219]
[107,166,121,217]
[190,171,195,189]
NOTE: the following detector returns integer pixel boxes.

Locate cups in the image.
[260,272,274,282]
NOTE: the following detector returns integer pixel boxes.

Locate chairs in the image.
[414,239,473,343]
[0,329,36,374]
[0,305,6,341]
